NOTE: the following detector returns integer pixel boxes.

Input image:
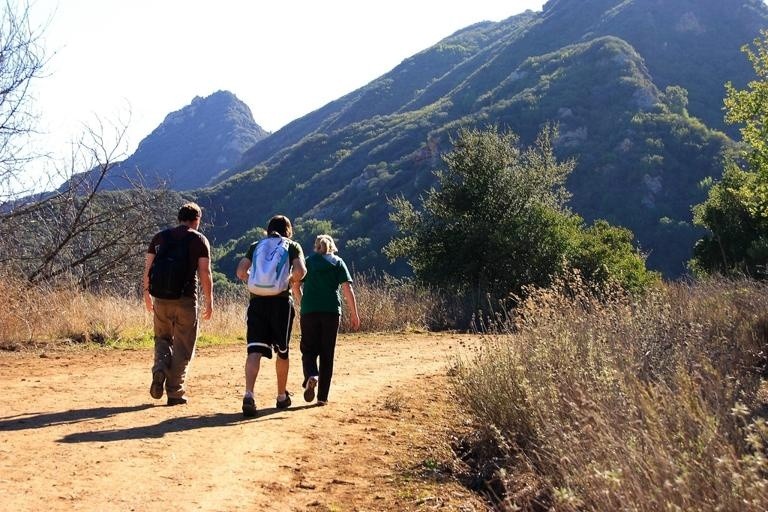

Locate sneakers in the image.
[304,376,328,406]
[150,369,166,399]
[277,391,294,410]
[167,397,187,405]
[242,397,256,417]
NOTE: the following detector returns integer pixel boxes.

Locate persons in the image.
[236,215,308,415]
[292,235,360,405]
[143,201,214,405]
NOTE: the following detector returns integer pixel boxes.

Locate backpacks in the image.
[149,228,202,299]
[247,231,291,296]
